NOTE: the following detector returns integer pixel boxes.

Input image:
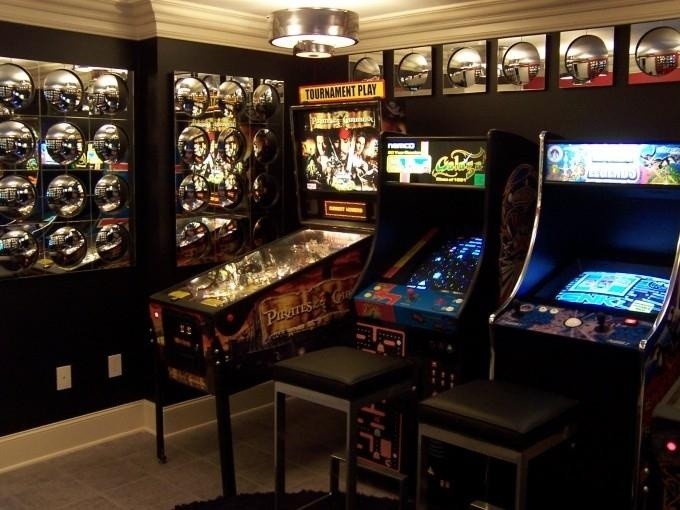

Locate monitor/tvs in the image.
[551,266,672,324]
[400,232,483,293]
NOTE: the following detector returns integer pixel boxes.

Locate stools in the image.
[272,343,418,509]
[417,375,577,507]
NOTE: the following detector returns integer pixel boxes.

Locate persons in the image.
[301,126,378,192]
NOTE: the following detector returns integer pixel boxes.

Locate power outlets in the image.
[47,352,144,397]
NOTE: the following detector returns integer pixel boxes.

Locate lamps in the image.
[269,2,359,57]
